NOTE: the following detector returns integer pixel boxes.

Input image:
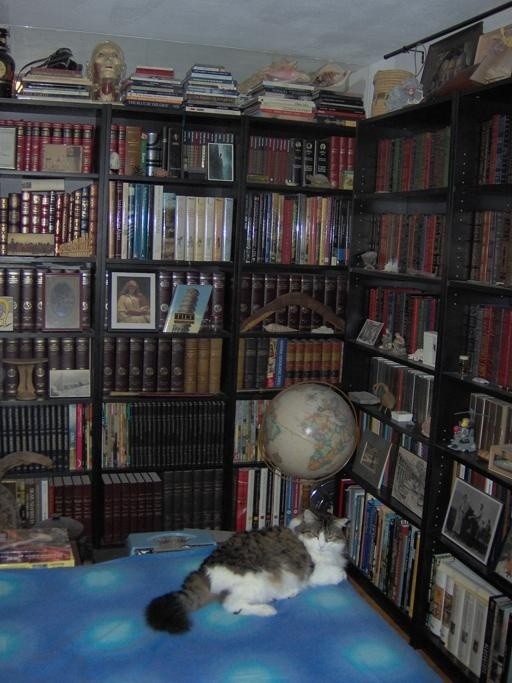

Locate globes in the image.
[259,380,360,514]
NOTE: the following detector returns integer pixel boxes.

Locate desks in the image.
[1,535,446,682]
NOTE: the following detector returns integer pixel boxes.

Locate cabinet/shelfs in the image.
[0,97,106,547]
[407,75,512,682]
[103,104,248,546]
[230,113,356,542]
[340,91,461,644]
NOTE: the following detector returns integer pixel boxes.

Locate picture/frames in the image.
[354,317,385,348]
[47,366,94,397]
[40,271,84,333]
[352,430,393,490]
[439,476,505,570]
[207,141,235,182]
[418,19,484,90]
[108,268,162,333]
[388,445,428,520]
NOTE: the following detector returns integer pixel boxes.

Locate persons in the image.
[86,38,130,103]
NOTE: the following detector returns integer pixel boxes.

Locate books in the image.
[108,180,233,262]
[235,467,334,532]
[365,285,511,392]
[377,112,511,195]
[233,399,274,462]
[16,67,94,103]
[242,191,351,267]
[1,262,91,330]
[363,211,511,289]
[236,337,343,390]
[1,337,89,397]
[1,118,93,174]
[102,402,224,468]
[110,124,234,183]
[102,470,225,542]
[1,403,94,473]
[356,410,512,565]
[246,135,354,190]
[180,64,240,115]
[240,270,347,334]
[1,176,96,257]
[104,269,225,332]
[338,478,511,681]
[1,529,82,570]
[238,80,367,127]
[103,336,222,394]
[120,65,184,109]
[2,476,91,536]
[368,355,511,460]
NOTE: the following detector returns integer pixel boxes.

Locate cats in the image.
[142,507,350,635]
